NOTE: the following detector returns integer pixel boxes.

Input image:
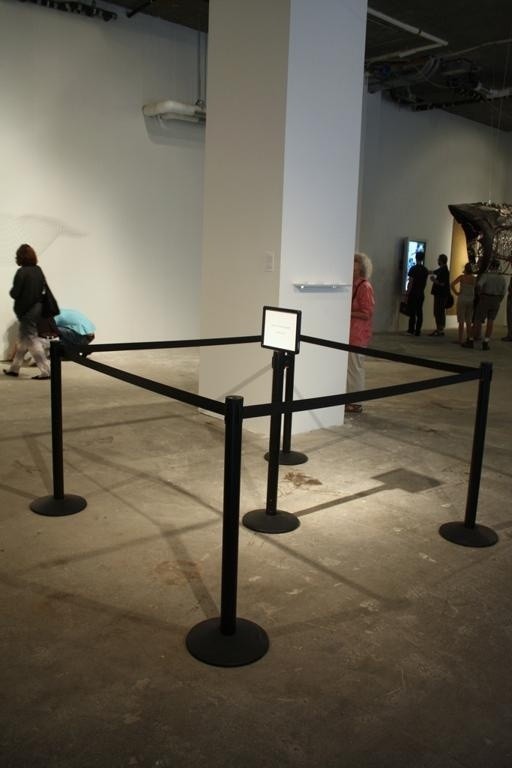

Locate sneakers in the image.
[3,369,19,376]
[345,404,362,413]
[32,375,51,379]
[401,331,412,336]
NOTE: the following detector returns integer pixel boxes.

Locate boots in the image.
[463,338,474,348]
[482,341,490,351]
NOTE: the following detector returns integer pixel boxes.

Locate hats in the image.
[463,263,478,274]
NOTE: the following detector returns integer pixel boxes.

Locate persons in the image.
[3,245,52,380]
[344,252,375,412]
[401,252,428,336]
[501,276,512,341]
[451,263,477,343]
[427,255,449,336]
[53,309,96,361]
[461,261,507,350]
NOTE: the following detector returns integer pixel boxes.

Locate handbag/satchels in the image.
[400,301,411,316]
[41,291,59,317]
